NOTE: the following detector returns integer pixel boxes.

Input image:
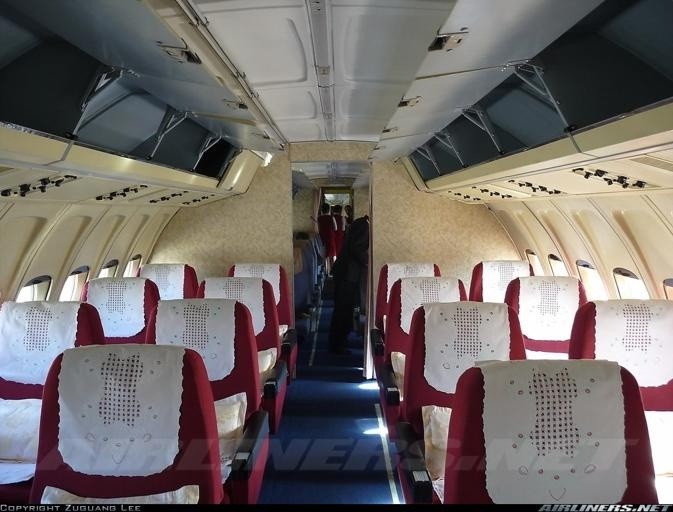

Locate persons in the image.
[317,202,337,279]
[325,214,370,356]
[344,204,354,227]
[329,205,344,261]
